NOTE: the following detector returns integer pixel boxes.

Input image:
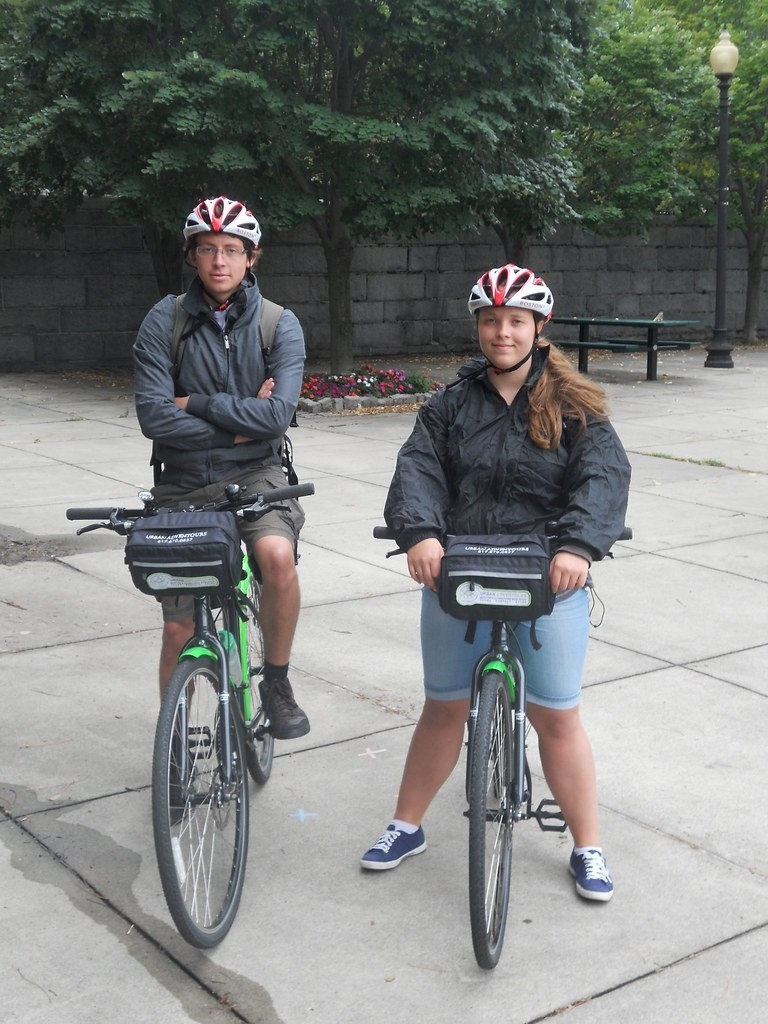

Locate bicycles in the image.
[64,483,321,948]
[370,523,635,969]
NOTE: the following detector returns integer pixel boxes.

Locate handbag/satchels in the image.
[126,508,244,596]
[438,533,555,622]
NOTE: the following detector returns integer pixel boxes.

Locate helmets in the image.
[468,264,555,325]
[182,195,262,248]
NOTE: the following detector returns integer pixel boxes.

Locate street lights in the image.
[704,30,741,369]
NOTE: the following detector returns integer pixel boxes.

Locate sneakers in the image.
[258,675,311,739]
[169,750,194,825]
[361,824,427,869]
[569,847,613,901]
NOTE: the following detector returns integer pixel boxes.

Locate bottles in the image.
[204,630,243,689]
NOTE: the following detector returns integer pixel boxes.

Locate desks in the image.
[550,313,700,383]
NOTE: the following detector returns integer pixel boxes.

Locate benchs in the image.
[549,334,701,353]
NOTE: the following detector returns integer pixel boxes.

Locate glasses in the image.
[193,243,248,260]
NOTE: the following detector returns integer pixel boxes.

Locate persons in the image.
[132,196,311,826]
[362,263,632,902]
